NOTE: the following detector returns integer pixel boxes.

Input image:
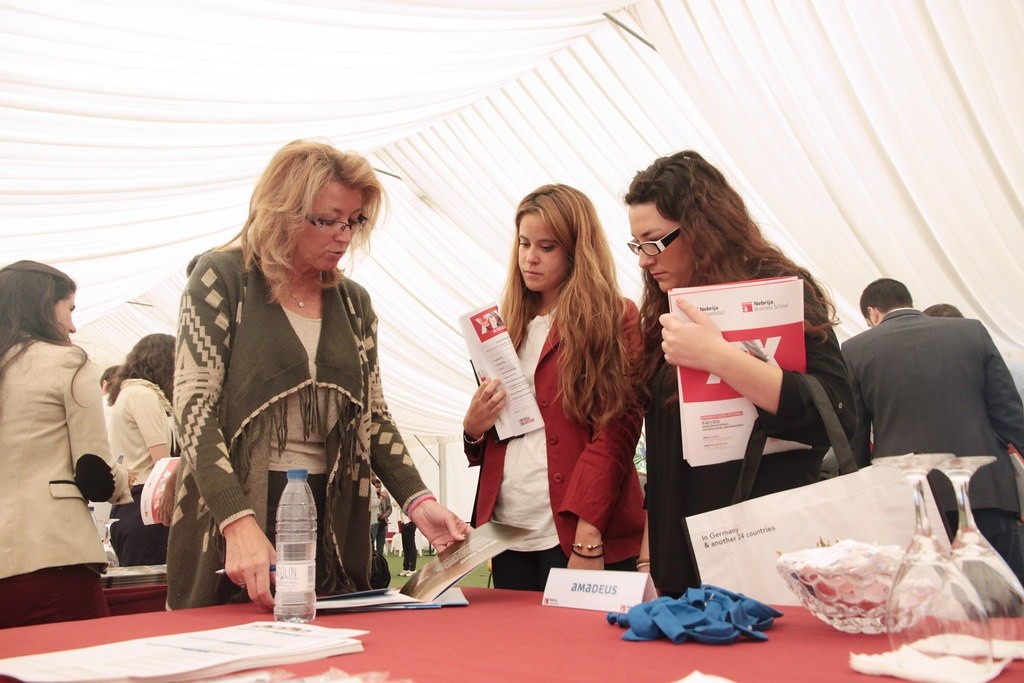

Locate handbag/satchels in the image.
[686,371,952,607]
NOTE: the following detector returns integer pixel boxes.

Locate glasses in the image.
[627,226,681,256]
[298,207,368,235]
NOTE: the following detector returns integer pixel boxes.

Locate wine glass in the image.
[932,455,1024,660]
[95,518,121,569]
[868,452,996,670]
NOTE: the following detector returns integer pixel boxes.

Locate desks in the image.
[0,586,1024,683]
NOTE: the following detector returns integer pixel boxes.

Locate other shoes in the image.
[405,570,417,576]
[399,570,408,576]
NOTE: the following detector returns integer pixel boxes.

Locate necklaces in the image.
[288,284,305,307]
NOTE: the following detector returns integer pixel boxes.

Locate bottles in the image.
[273,467,318,623]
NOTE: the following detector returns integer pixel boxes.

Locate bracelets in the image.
[463,433,484,444]
[635,559,650,568]
[408,494,436,519]
[572,541,603,548]
[571,547,603,558]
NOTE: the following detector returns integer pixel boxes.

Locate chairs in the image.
[390,528,424,557]
[93,581,169,618]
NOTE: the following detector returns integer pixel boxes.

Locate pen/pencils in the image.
[214,565,277,575]
[477,370,504,424]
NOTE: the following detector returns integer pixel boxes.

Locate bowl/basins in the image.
[778,561,922,635]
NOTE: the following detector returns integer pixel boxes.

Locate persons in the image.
[366,473,419,576]
[819,279,1024,586]
[100,332,175,568]
[626,151,856,600]
[0,259,138,630]
[462,183,646,591]
[163,140,476,612]
